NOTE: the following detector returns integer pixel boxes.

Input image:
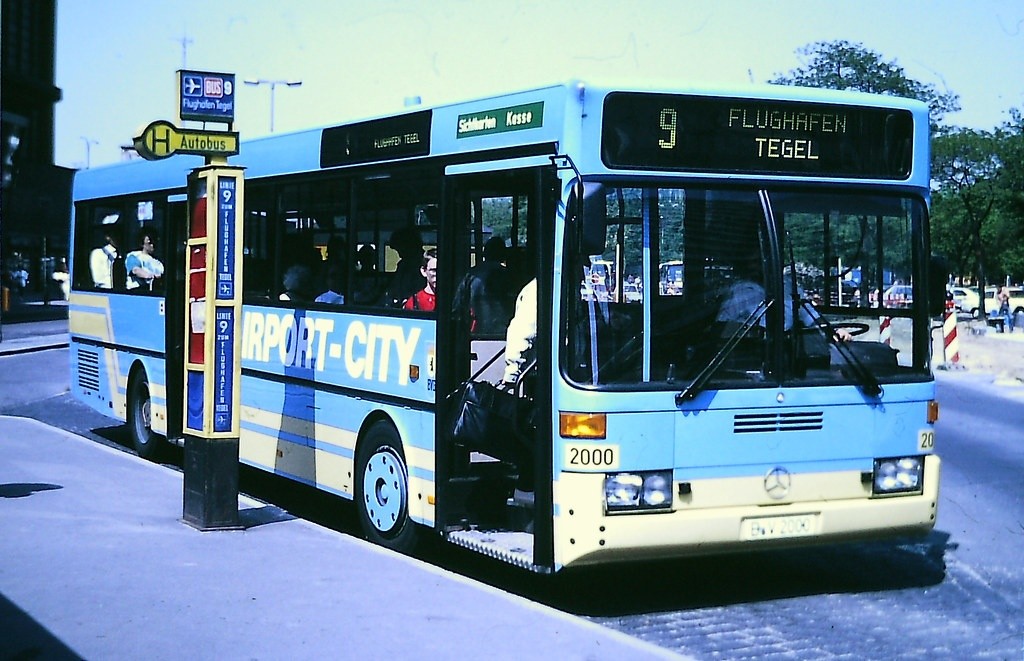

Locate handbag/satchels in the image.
[451,379,532,464]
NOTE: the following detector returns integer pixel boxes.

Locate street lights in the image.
[243,79,303,134]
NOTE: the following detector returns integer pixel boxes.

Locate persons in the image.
[716,242,830,339]
[90,224,119,289]
[404,250,477,334]
[995,284,1014,334]
[280,229,427,305]
[453,236,531,338]
[125,226,164,290]
[500,252,590,504]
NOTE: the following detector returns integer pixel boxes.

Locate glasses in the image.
[426,267,437,275]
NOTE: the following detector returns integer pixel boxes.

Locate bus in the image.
[67,78,942,576]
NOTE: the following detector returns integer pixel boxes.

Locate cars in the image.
[580,259,1024,328]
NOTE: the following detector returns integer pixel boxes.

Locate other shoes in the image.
[513,489,534,508]
